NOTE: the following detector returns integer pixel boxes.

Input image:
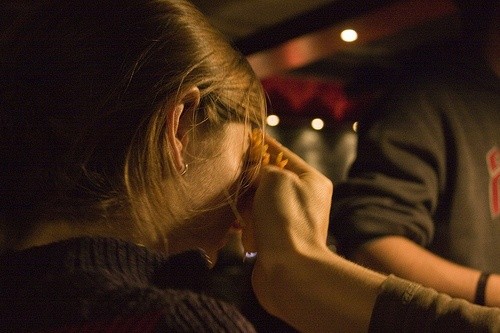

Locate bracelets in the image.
[474,271,491,305]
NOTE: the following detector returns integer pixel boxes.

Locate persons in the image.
[328,0,499,310]
[0,0,500,333]
